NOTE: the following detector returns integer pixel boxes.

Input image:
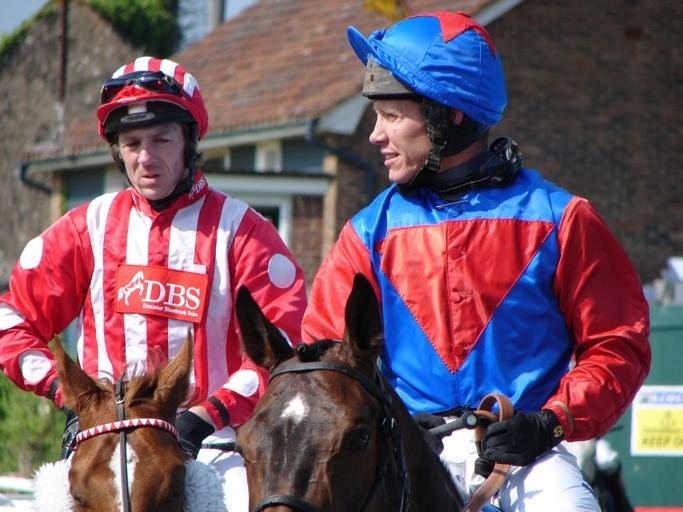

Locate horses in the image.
[51,325,191,512]
[234,271,462,512]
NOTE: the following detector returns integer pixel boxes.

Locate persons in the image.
[593,437,634,512]
[295,7,653,511]
[0,54,311,511]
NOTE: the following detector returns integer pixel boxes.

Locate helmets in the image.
[346,10,509,158]
[96,56,208,169]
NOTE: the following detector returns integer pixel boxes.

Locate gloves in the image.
[481,408,564,467]
[60,408,81,460]
[173,409,215,460]
